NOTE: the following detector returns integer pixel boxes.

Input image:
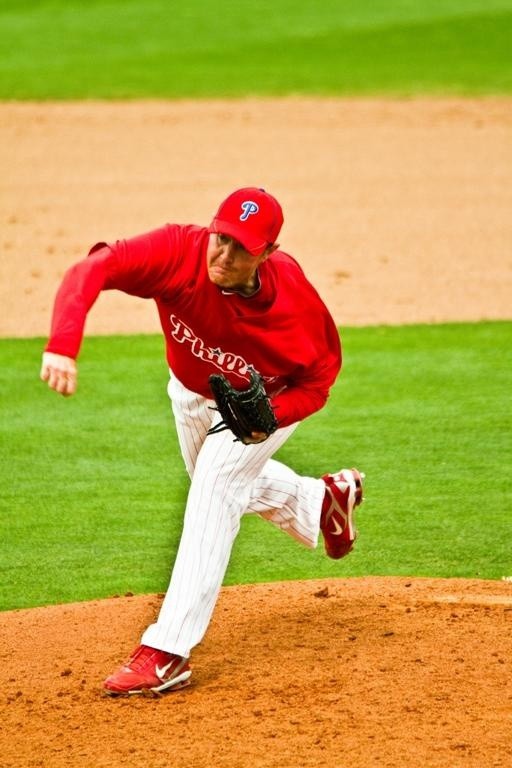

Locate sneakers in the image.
[105,646,191,697]
[320,470,363,561]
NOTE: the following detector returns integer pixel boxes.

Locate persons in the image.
[37,185,365,700]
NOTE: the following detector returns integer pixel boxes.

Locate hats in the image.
[208,187,285,256]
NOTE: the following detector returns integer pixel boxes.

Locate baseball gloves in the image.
[209,367,277,444]
